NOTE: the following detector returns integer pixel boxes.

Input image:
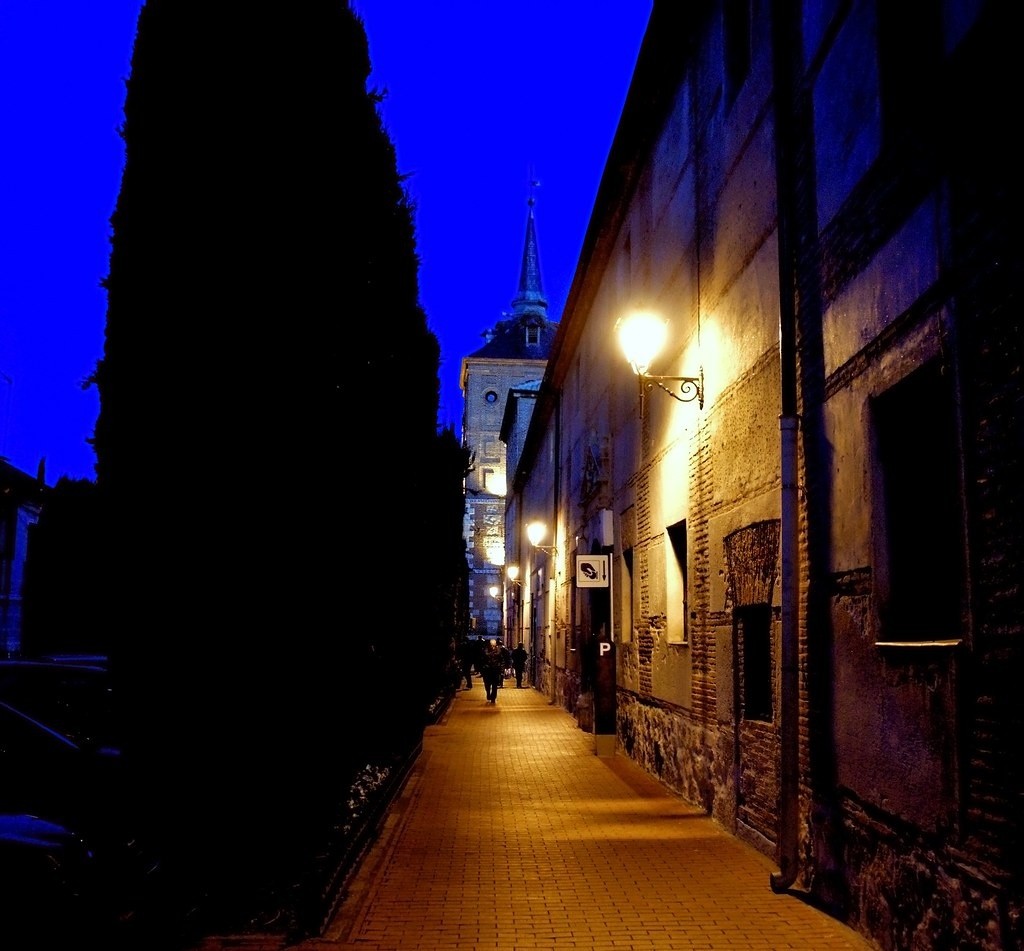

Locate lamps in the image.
[618,313,705,420]
[527,522,558,558]
[490,567,525,602]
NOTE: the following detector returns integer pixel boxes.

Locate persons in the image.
[458,635,527,706]
